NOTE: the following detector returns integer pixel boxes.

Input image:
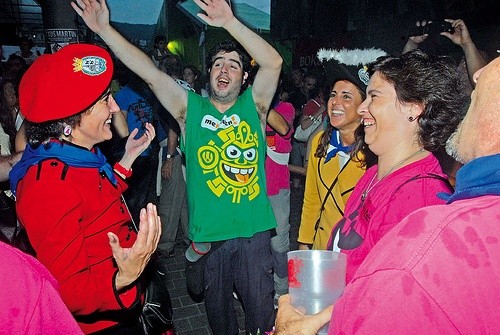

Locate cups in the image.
[185,242,212,262]
[286,249,347,334]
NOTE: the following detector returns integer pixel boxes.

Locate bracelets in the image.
[165,154,175,159]
[114,162,133,178]
[308,114,317,122]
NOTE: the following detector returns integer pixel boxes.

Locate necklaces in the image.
[361,146,424,201]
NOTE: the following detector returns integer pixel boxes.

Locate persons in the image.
[0,35,211,260]
[16,44,176,335]
[70,0,284,335]
[241,0,500,335]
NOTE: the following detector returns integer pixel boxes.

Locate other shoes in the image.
[155,248,175,256]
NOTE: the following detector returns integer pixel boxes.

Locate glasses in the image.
[305,82,315,86]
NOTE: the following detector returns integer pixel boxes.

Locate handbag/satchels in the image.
[138,260,173,335]
[187,257,207,303]
[293,114,322,142]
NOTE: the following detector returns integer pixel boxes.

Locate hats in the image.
[317,32,389,93]
[19,44,114,122]
